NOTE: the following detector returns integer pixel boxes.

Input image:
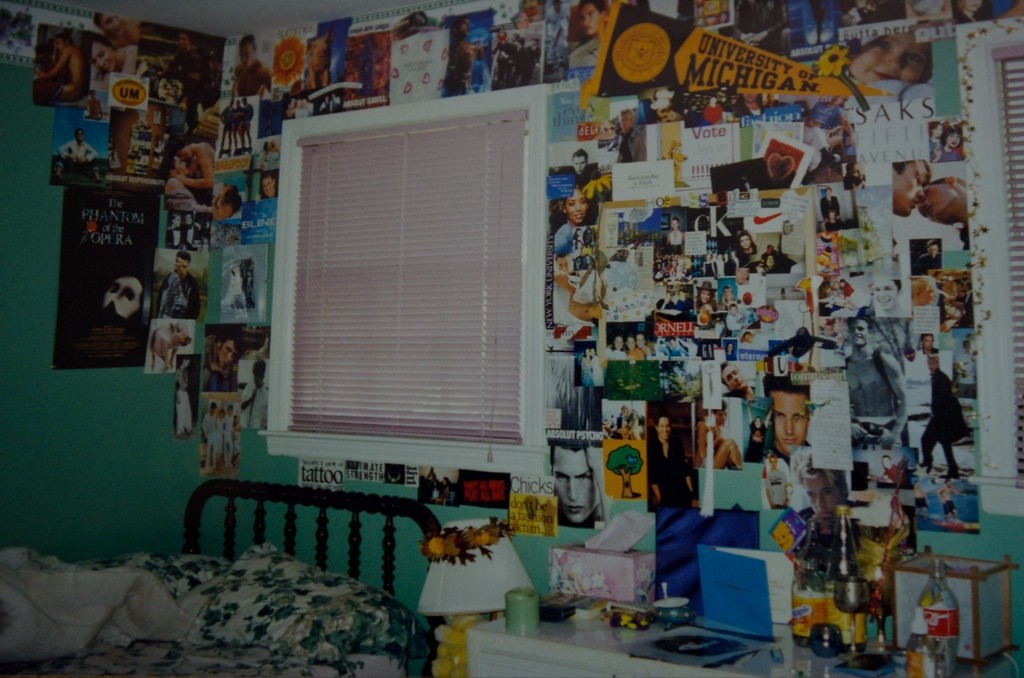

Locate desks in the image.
[467,613,850,677]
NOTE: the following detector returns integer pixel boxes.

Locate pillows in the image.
[183,542,432,678]
[74,551,233,599]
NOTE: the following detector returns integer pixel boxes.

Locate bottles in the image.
[907,559,959,678]
[67,147,76,160]
[830,351,846,366]
[571,246,595,304]
[771,481,786,505]
[791,504,867,651]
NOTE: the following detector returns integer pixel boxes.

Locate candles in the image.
[505,586,540,630]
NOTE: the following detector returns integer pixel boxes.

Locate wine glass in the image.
[832,576,870,660]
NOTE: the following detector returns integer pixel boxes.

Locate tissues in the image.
[549,509,656,605]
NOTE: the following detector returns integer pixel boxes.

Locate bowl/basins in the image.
[893,651,907,664]
[652,598,689,618]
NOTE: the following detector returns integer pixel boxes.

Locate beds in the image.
[0,479,443,678]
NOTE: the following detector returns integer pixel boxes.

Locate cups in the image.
[505,588,540,629]
[811,623,842,657]
[553,323,566,339]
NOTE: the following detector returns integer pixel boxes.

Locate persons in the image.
[220,96,253,155]
[166,137,215,206]
[87,29,138,79]
[283,92,340,118]
[165,157,212,211]
[203,403,238,470]
[241,360,268,429]
[177,360,195,437]
[94,13,141,49]
[392,0,1024,529]
[54,127,103,183]
[36,33,87,102]
[172,33,212,111]
[159,252,200,320]
[212,183,243,220]
[232,36,270,97]
[211,335,239,393]
[166,213,209,250]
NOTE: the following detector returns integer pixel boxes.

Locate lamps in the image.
[889,545,1019,672]
[416,519,536,616]
